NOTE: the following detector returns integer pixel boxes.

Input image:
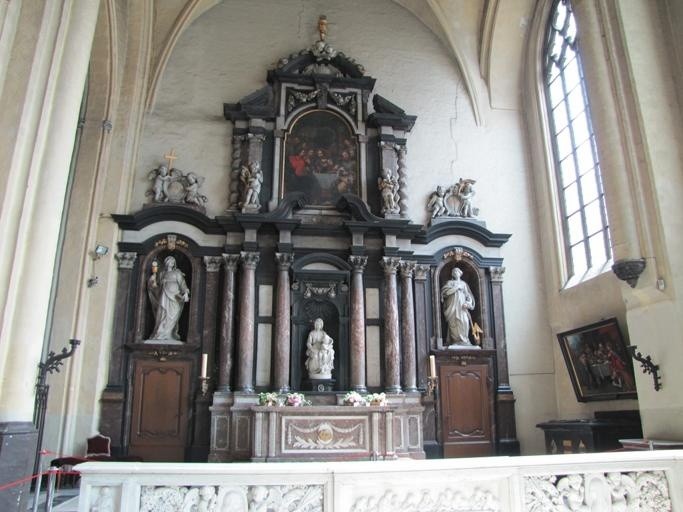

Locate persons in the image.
[441,267,476,345]
[152,166,170,202]
[148,255,190,340]
[183,172,204,206]
[578,340,627,388]
[430,184,446,219]
[460,183,474,218]
[377,168,394,210]
[304,318,335,374]
[287,135,357,190]
[244,161,264,206]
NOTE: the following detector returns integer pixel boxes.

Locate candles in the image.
[429,354,436,377]
[201,353,208,377]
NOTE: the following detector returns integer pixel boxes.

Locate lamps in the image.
[610,255,647,289]
[38,337,82,397]
[87,244,109,287]
[625,345,662,392]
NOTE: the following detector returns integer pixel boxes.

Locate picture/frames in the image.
[555,317,638,403]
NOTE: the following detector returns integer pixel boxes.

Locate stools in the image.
[50,455,95,489]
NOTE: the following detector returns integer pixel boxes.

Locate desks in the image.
[534,417,622,454]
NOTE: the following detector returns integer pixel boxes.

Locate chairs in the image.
[84,433,111,462]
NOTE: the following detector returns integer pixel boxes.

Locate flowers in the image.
[257,390,313,408]
[343,389,388,407]
